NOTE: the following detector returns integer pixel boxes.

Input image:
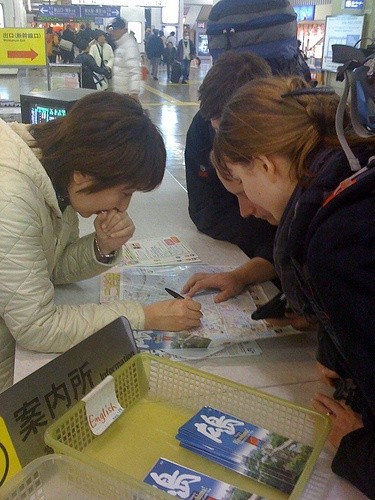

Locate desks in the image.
[11,167,371,500]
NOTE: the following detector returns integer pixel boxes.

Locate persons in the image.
[77,16,144,101]
[0,91,202,392]
[206,0,317,89]
[145,27,195,83]
[45,23,117,64]
[177,51,375,500]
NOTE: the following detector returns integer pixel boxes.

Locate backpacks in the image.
[206,0,313,83]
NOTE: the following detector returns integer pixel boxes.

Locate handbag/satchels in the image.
[58,38,74,52]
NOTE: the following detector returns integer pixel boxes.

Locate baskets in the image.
[0,453,180,500]
[44,353,332,500]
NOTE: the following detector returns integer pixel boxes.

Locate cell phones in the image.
[251,292,292,321]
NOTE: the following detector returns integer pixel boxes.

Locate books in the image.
[134,458,265,500]
[176,406,314,495]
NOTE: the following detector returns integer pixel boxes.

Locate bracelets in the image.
[94,239,118,260]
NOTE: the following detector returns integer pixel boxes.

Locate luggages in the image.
[170,63,182,83]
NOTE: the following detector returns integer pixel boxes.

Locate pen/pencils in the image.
[165,288,203,315]
